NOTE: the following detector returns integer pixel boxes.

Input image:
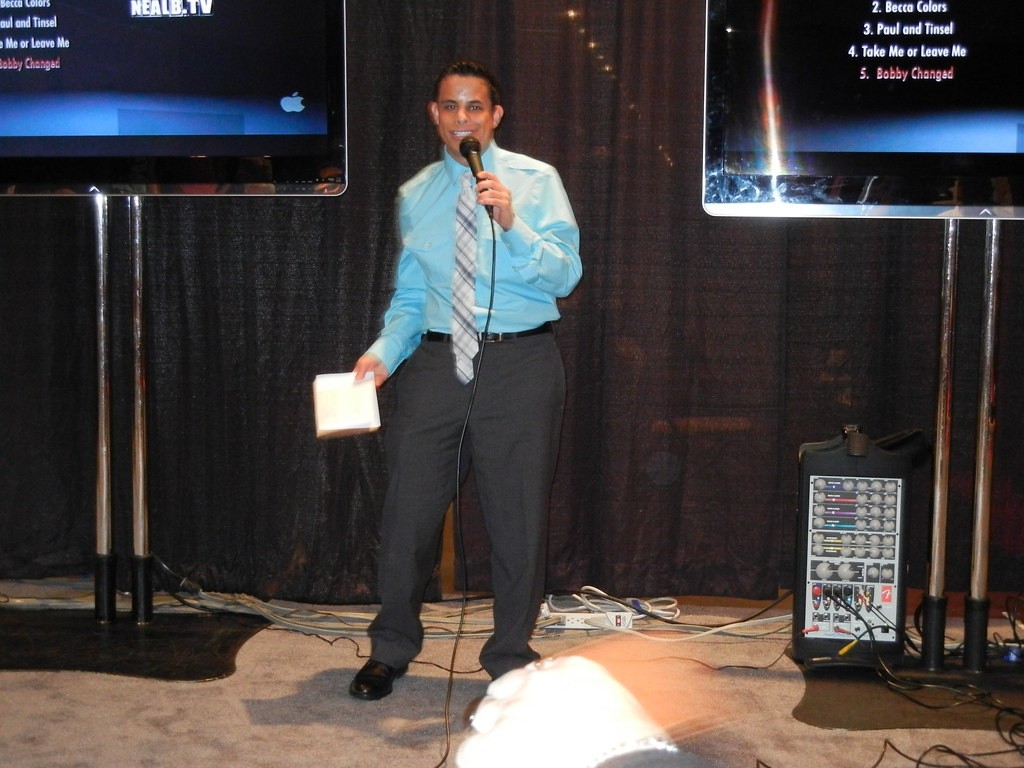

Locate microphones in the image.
[460,136,493,218]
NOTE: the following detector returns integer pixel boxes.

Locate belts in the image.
[420,321,554,343]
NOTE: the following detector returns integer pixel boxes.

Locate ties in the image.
[452,172,479,385]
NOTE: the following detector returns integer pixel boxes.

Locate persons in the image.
[348,62,584,701]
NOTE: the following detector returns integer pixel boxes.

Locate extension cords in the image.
[542,613,635,631]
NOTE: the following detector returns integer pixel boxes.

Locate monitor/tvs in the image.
[0,0,348,197]
[702,0,1024,221]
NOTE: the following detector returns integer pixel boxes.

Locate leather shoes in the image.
[349,657,397,698]
